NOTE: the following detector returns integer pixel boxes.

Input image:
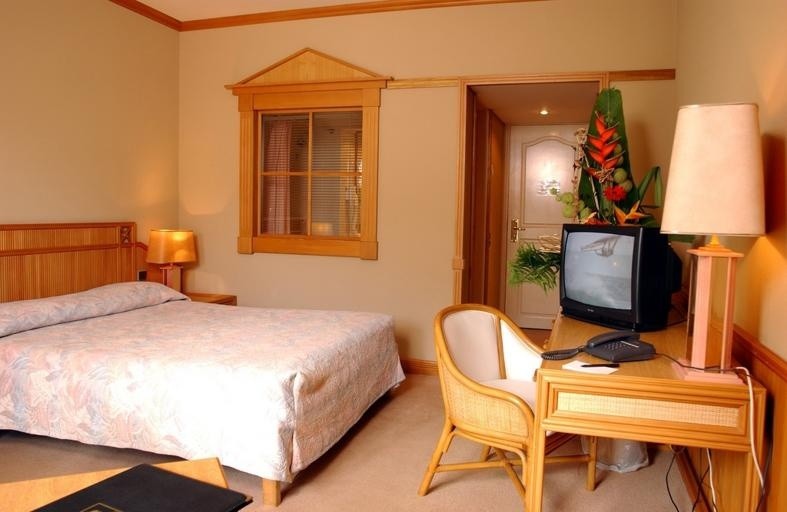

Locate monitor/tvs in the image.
[559,223,683,333]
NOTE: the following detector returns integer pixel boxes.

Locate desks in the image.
[0,456,235,512]
[523,305,768,512]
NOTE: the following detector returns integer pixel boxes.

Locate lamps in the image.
[659,102,766,385]
[144,227,199,294]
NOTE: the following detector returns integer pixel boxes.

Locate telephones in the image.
[583,330,656,362]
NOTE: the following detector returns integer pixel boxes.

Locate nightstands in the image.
[180,291,238,307]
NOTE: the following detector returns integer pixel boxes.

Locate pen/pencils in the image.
[581,363,619,367]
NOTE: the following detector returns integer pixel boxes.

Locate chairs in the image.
[416,301,599,511]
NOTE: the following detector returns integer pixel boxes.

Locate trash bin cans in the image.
[580,434,649,472]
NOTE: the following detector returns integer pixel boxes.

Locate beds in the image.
[0,220,407,508]
